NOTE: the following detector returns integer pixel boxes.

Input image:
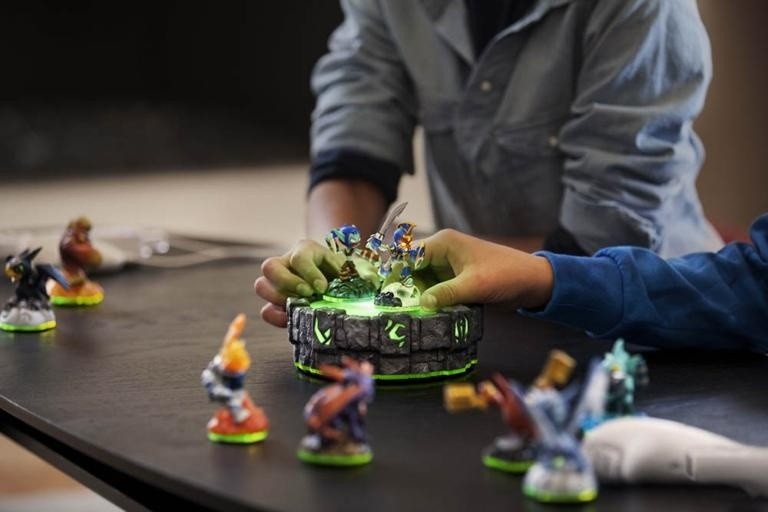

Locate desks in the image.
[0,235,759,511]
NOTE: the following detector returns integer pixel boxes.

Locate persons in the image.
[409,212,768,360]
[254,0,726,330]
